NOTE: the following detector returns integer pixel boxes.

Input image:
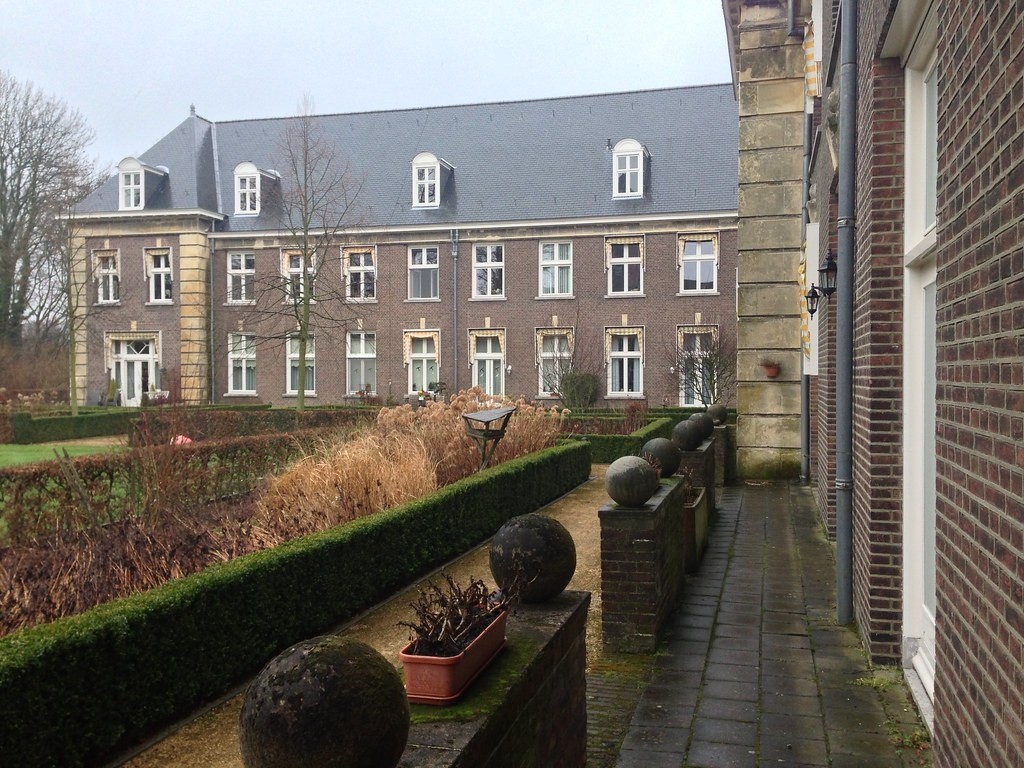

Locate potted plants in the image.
[760,359,782,376]
[397,572,524,707]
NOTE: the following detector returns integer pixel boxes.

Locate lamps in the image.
[817,244,837,303]
[804,283,826,320]
